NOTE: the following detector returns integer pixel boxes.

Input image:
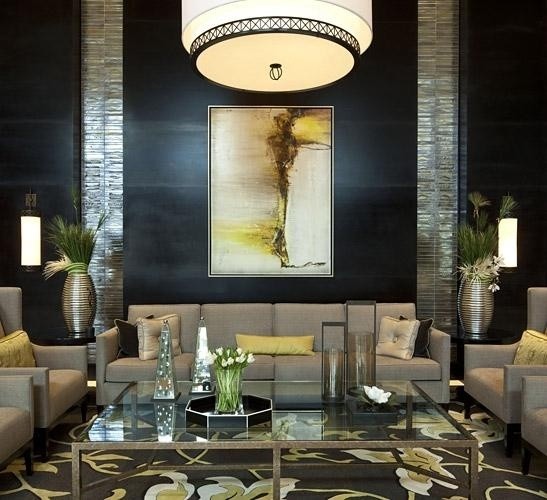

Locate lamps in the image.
[180,0,374,93]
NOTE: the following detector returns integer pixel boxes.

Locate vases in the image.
[458,277,494,335]
[214,367,244,414]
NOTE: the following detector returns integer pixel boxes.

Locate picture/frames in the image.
[206,104,335,278]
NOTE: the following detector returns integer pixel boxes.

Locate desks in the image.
[443,326,514,420]
[34,329,96,424]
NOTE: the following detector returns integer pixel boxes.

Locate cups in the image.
[349,330,375,391]
[324,347,348,402]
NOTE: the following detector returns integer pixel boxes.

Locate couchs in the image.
[96,303,451,421]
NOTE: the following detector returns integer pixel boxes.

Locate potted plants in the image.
[42,212,109,338]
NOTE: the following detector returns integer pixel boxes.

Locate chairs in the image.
[0,375,34,475]
[520,376,547,476]
[464,287,547,457]
[0,287,88,459]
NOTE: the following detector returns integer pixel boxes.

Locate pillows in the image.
[0,329,37,368]
[513,330,547,365]
[399,315,433,358]
[235,334,315,356]
[375,316,420,360]
[114,314,153,359]
[137,314,182,361]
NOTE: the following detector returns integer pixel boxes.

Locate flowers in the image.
[202,347,255,367]
[364,385,391,404]
[452,192,515,293]
